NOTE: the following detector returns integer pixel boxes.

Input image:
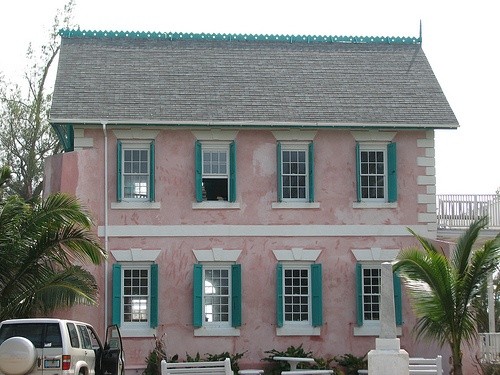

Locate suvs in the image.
[0,317,125,375]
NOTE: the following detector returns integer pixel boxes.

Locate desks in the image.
[272,355,315,372]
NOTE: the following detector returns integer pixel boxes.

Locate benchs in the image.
[280,369,335,375]
[409,355,444,374]
[160,356,234,375]
[238,369,265,375]
